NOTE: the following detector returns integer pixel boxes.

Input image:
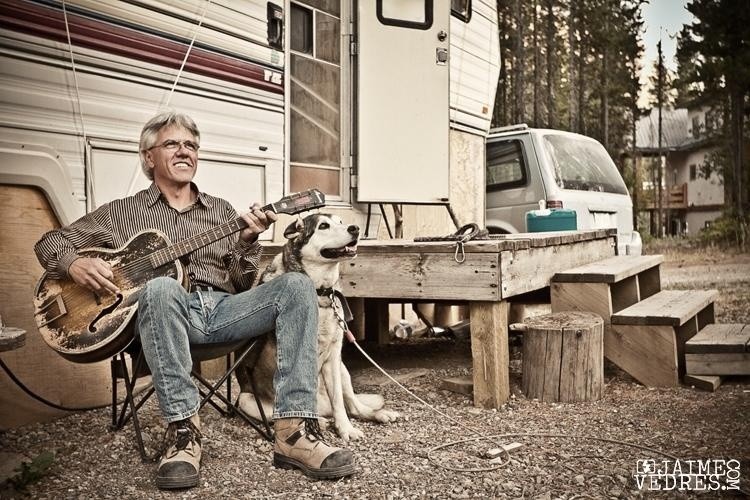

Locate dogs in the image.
[232,212,400,442]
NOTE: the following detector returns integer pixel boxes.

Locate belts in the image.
[191,284,218,291]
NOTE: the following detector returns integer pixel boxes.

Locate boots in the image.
[155,413,203,489]
[273,418,354,479]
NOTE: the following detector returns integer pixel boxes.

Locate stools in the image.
[106,325,275,463]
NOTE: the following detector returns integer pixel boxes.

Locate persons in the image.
[31,111,361,491]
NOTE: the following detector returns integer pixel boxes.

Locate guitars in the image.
[30,188,326,364]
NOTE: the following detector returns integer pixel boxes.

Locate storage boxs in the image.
[524,207,578,233]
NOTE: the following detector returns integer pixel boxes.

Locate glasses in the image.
[149,140,200,151]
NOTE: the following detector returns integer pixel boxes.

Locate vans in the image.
[486,124,642,255]
